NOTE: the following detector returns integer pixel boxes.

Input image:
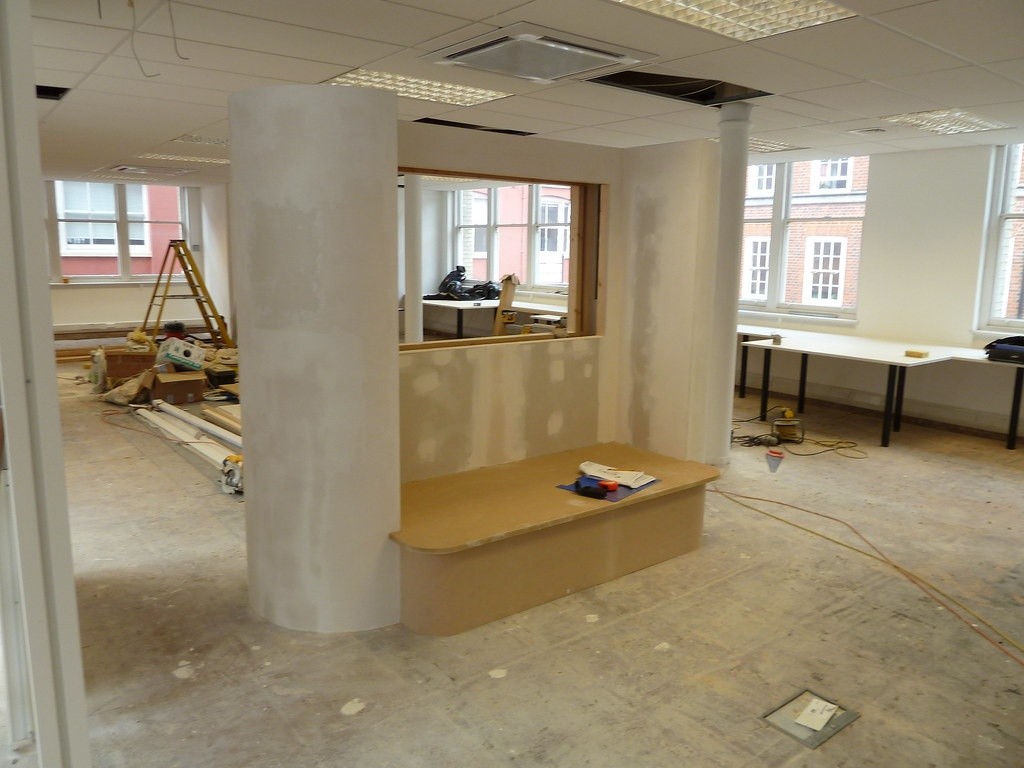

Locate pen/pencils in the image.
[608,469,635,471]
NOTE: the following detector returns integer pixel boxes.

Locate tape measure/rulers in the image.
[598,479,618,491]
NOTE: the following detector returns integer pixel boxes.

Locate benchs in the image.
[389,442,723,636]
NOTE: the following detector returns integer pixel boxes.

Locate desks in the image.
[421,293,569,340]
[734,324,1024,449]
[528,314,562,326]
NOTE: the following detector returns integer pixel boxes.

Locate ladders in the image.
[142,239,232,348]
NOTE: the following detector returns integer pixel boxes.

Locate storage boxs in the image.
[101,337,237,406]
